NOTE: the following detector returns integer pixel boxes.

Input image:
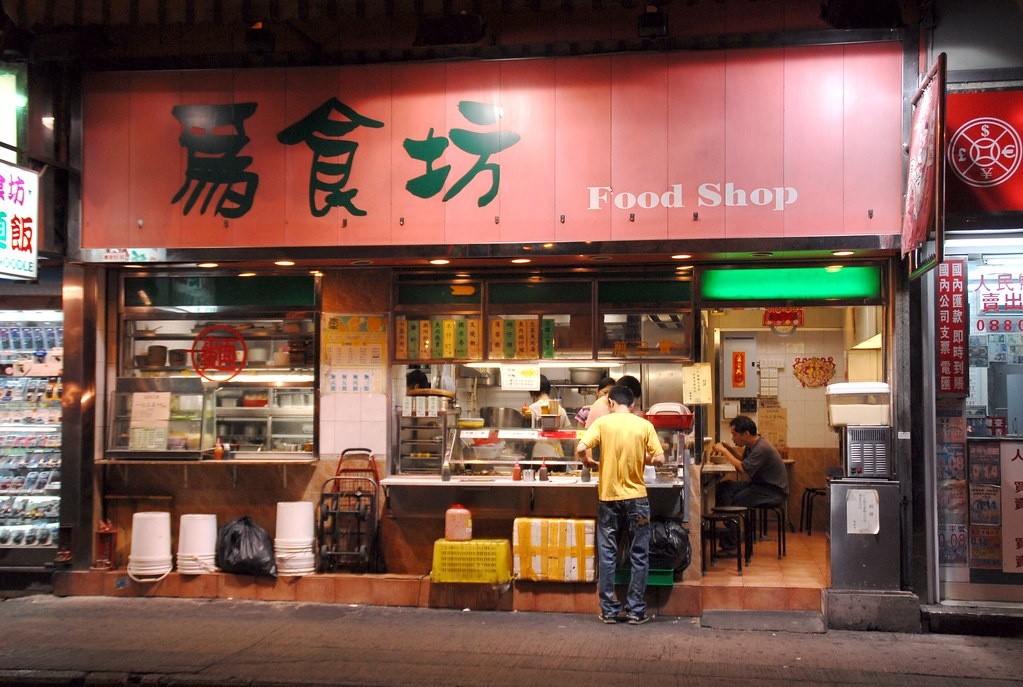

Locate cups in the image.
[523,469,535,481]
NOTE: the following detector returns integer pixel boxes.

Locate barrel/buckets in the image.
[176,513,217,575]
[127,512,173,583]
[273,501,316,577]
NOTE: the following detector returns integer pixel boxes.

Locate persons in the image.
[524,375,572,473]
[574,374,642,472]
[576,385,665,624]
[406,369,428,391]
[714,415,789,559]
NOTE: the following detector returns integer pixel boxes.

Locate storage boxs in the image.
[430,539,513,583]
[513,517,596,582]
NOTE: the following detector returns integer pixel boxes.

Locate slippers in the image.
[705,527,730,537]
[715,548,738,558]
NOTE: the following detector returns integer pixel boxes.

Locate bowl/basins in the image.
[147,345,167,367]
[242,400,268,407]
[711,455,728,464]
[168,349,187,367]
[247,348,268,362]
[274,352,289,366]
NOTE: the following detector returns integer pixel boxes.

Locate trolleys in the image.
[317,476,381,575]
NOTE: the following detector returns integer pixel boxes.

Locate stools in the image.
[746,504,786,559]
[701,513,743,576]
[710,506,752,566]
[798,488,826,536]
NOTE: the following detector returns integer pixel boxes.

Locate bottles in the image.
[214,437,224,460]
[524,407,532,428]
[223,443,231,460]
[445,504,472,542]
[513,457,521,480]
[521,402,528,427]
[441,460,450,481]
[581,465,590,482]
[539,457,548,481]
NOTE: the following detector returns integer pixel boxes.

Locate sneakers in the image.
[629,615,650,625]
[599,611,617,624]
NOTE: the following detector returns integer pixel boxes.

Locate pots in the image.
[568,367,604,384]
